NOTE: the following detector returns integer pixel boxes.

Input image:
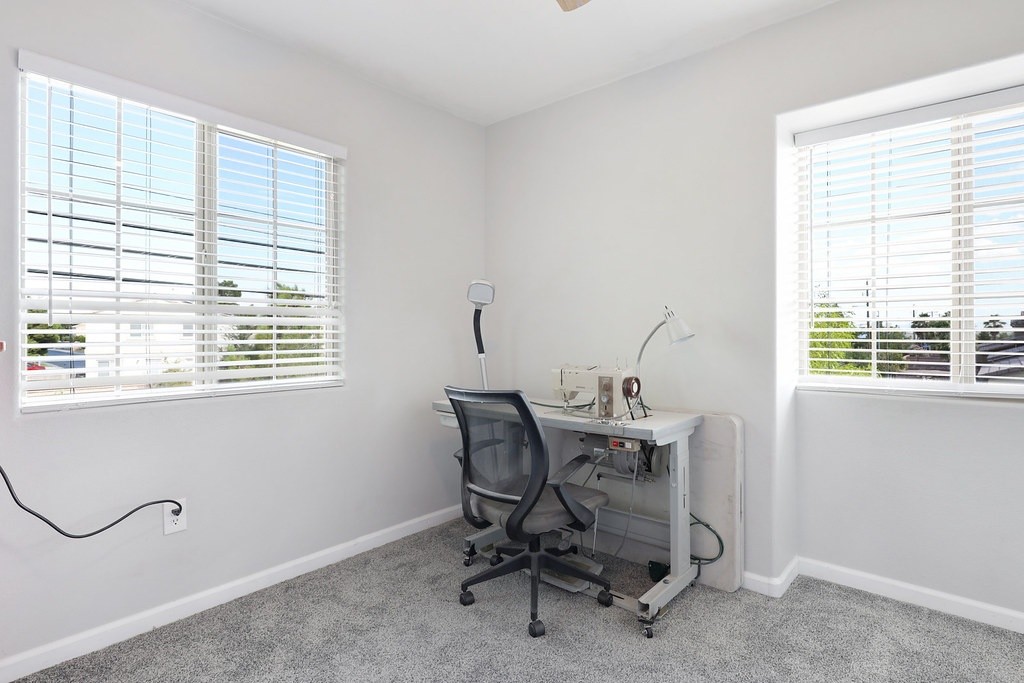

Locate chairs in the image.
[443,385,613,639]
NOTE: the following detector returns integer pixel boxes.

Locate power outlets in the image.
[162,498,187,536]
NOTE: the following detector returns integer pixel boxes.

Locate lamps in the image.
[466,279,500,485]
[635,304,696,418]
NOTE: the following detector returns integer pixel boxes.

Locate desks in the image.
[432,397,706,638]
